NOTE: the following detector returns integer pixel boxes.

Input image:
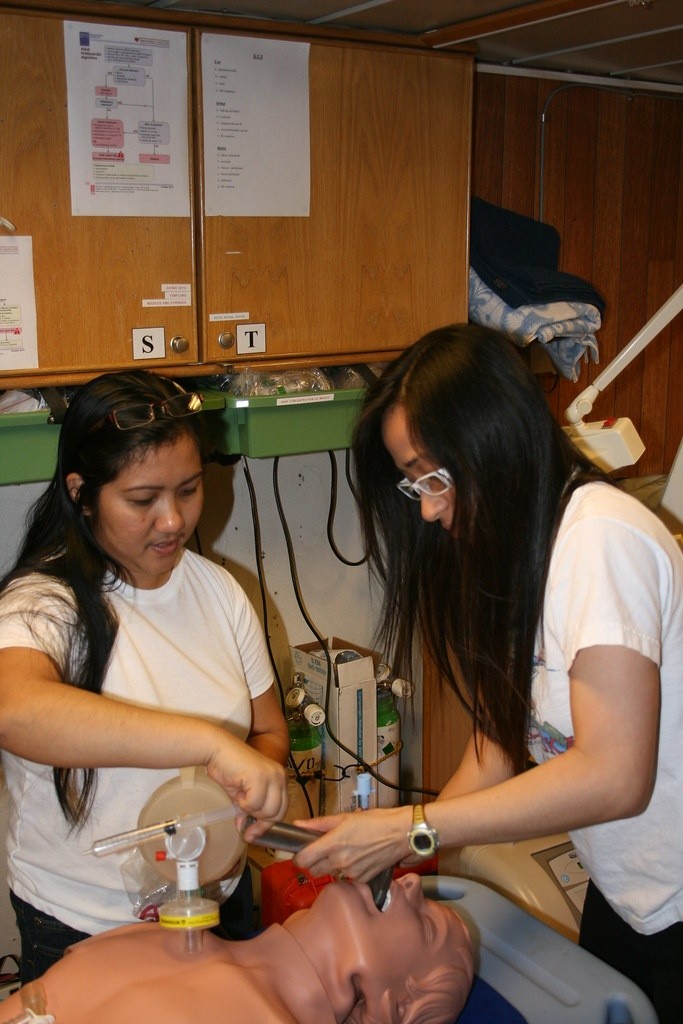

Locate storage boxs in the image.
[176,362,401,458]
[286,637,377,817]
[1,377,225,483]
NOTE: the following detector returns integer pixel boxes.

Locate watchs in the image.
[406,805,440,860]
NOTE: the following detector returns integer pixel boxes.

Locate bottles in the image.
[272,719,321,825]
[376,690,401,808]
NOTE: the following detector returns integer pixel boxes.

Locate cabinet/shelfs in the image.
[0,0,477,387]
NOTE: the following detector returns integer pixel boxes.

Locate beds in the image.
[379,874,660,1024]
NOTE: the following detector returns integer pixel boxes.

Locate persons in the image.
[292,322,683,1024]
[0,366,290,987]
[0,873,473,1024]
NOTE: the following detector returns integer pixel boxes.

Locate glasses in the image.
[84,392,204,435]
[397,468,453,501]
